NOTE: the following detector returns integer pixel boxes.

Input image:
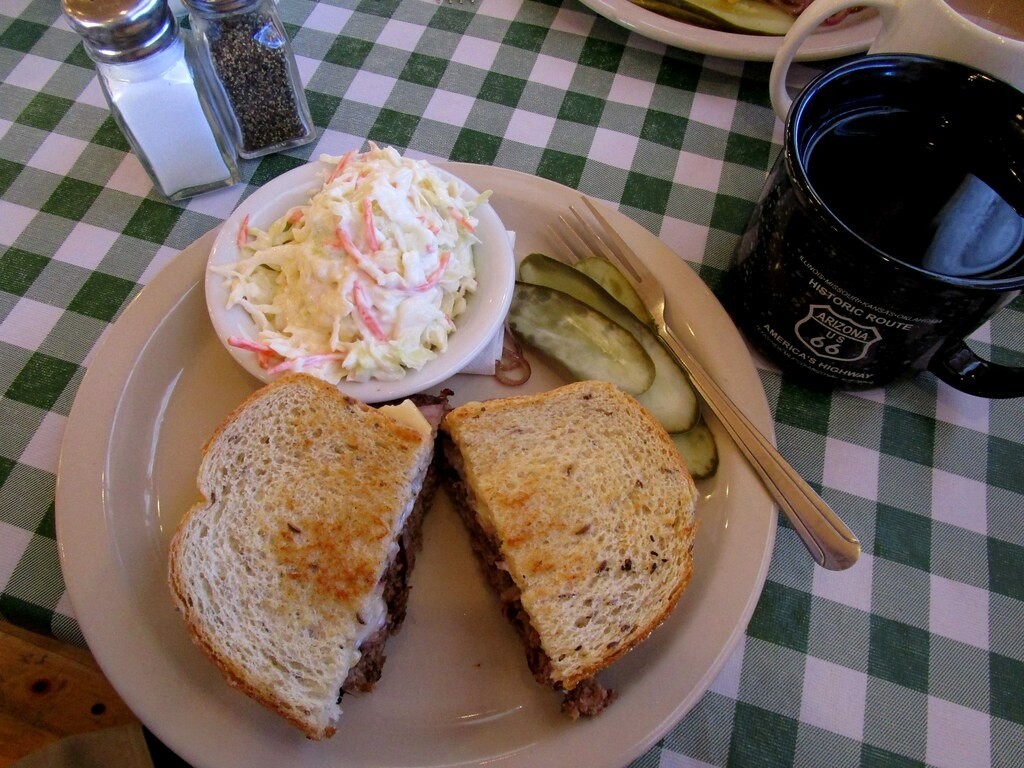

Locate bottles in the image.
[61,1,241,202]
[181,0,314,159]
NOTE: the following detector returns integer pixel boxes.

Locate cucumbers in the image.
[504,254,719,481]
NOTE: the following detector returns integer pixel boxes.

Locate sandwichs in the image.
[163,372,702,742]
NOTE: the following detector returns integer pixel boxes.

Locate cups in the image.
[721,52,1024,398]
[769,0,1022,123]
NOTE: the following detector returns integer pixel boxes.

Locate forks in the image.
[541,194,862,572]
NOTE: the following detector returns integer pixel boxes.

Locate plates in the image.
[579,0,880,63]
[57,158,775,767]
[205,153,517,403]
